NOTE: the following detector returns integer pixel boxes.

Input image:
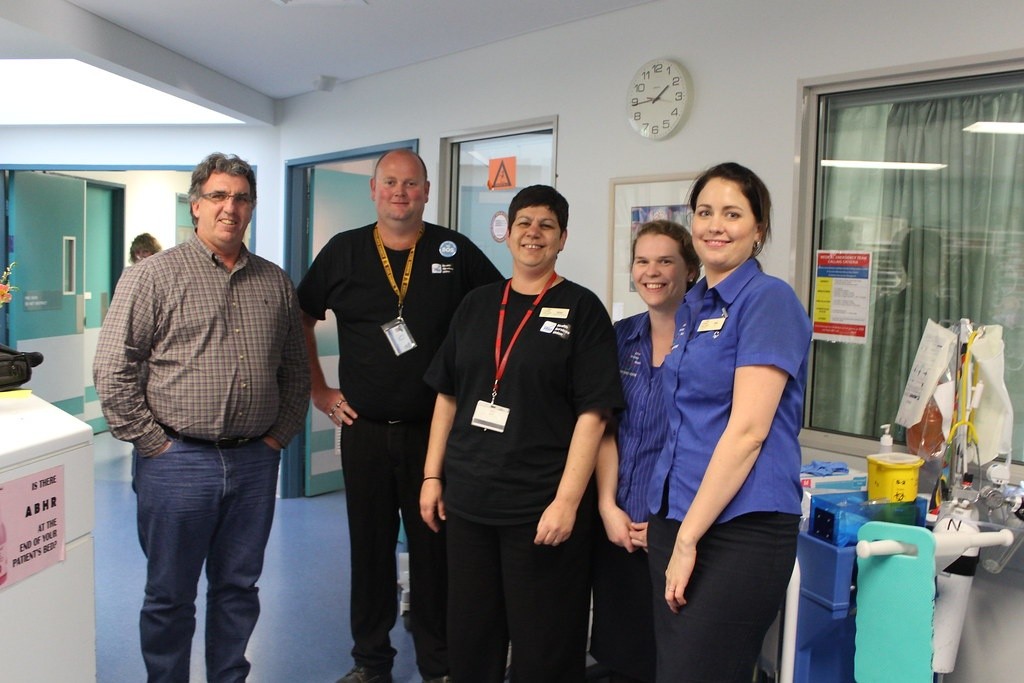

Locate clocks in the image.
[624,57,692,141]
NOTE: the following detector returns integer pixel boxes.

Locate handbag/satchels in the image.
[970,324,1014,466]
[895,318,960,429]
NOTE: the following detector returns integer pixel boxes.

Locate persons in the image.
[419,185,630,683]
[644,161,815,683]
[588,217,700,683]
[127,233,162,263]
[91,153,312,683]
[291,148,509,683]
[867,225,1000,443]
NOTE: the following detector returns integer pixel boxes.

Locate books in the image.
[0,388,33,400]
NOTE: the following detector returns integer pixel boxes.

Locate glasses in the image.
[199,191,252,206]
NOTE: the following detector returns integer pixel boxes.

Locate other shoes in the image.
[335,666,393,683]
[422,675,448,683]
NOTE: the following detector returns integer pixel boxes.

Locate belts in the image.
[358,414,429,425]
[154,419,271,449]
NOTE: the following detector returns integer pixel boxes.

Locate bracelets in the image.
[421,476,443,483]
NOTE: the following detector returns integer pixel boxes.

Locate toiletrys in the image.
[880,424,893,453]
[950,473,974,518]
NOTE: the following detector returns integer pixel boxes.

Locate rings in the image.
[328,412,333,418]
[336,400,343,407]
